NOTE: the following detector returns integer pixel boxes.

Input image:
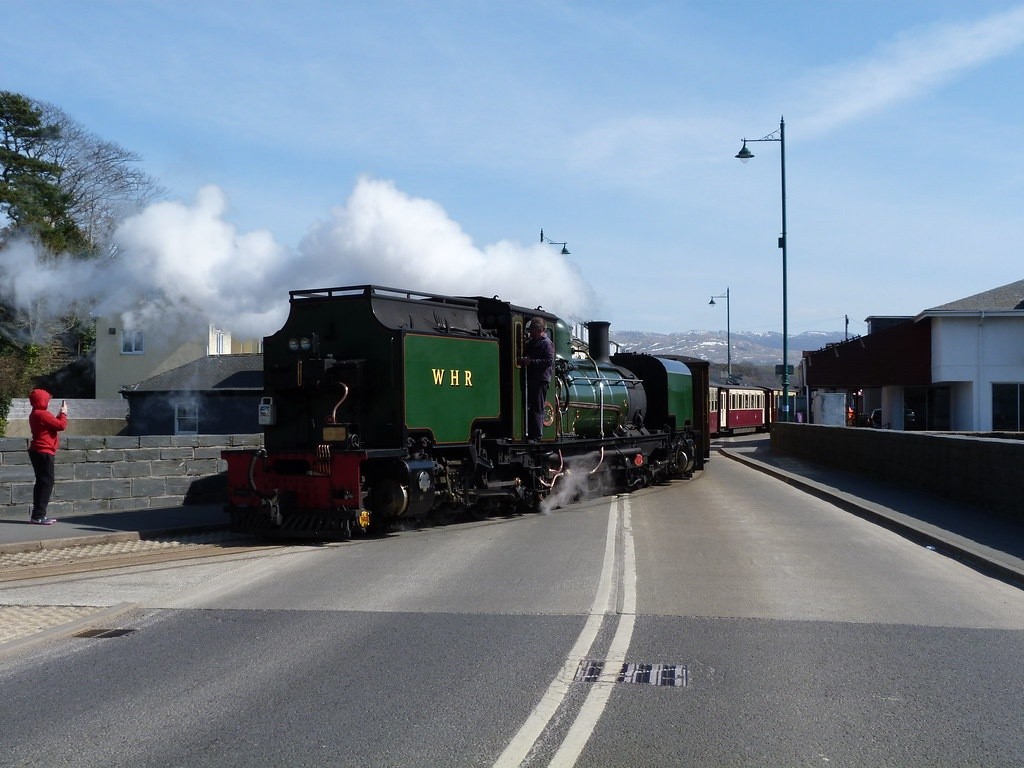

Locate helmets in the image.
[530,318,547,330]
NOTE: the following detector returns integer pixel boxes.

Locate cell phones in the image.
[62,400,66,406]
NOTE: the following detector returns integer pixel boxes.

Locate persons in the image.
[520,317,555,444]
[28,389,67,524]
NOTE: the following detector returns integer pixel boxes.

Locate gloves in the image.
[522,357,531,365]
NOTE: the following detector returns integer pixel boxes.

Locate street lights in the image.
[734,116,790,421]
[708,288,732,378]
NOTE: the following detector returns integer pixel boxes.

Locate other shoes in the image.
[527,437,541,445]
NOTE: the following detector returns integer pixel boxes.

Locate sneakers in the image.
[31,517,57,524]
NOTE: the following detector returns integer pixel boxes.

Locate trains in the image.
[220,283,711,545]
